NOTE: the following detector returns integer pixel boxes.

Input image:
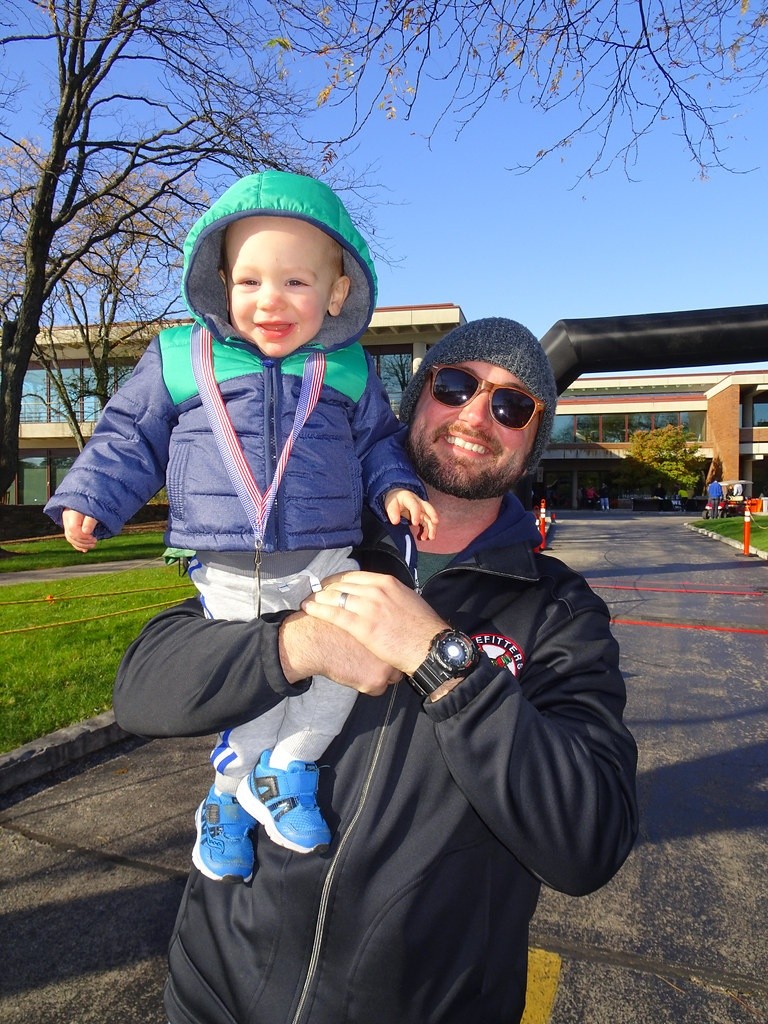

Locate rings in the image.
[338,593,350,609]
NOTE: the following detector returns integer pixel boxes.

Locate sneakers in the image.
[236,749,331,855]
[192,784,257,885]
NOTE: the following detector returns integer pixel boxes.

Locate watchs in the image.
[407,626,479,698]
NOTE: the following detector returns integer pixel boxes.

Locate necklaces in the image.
[192,321,324,617]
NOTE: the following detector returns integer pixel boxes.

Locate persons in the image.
[110,316,636,1024]
[546,487,553,506]
[577,481,609,510]
[45,169,440,884]
[672,483,681,495]
[531,487,535,508]
[726,484,734,499]
[708,477,723,519]
[654,483,666,499]
[678,490,688,512]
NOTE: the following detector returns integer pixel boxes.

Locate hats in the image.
[400,318,558,476]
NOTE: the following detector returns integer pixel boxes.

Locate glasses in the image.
[428,364,545,431]
